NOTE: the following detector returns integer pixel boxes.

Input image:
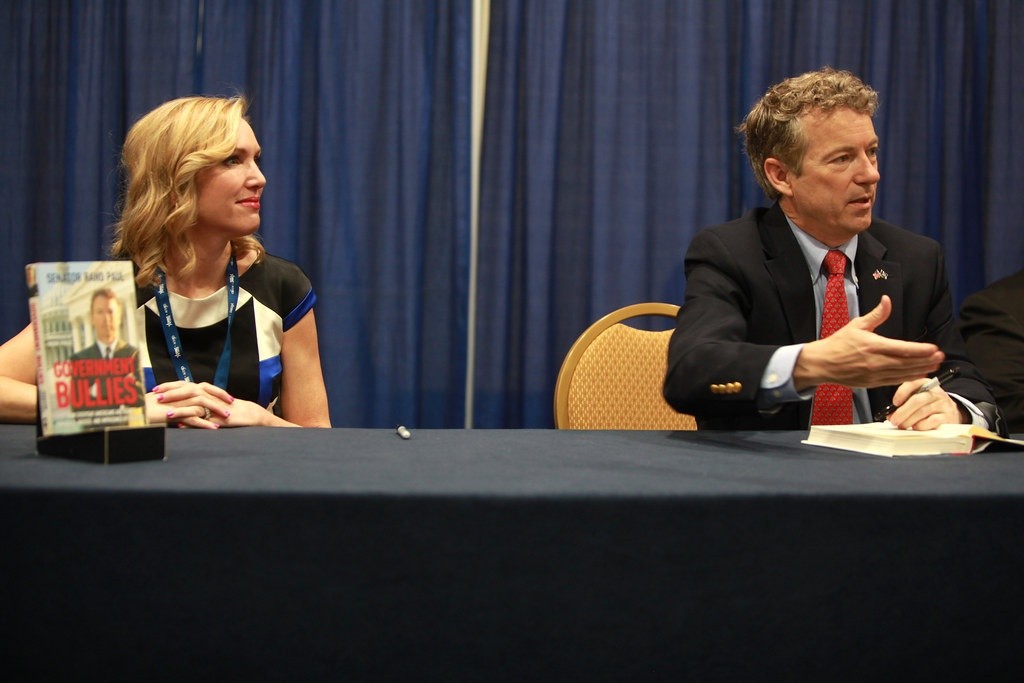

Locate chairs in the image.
[554,301,698,434]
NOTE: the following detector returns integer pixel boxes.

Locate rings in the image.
[203,407,210,420]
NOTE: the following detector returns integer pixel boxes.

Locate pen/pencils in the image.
[394,423,412,440]
[873,365,964,422]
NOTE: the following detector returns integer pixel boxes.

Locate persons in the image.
[662,67,1010,438]
[0,95,330,430]
[70,288,144,431]
[960,268,1024,433]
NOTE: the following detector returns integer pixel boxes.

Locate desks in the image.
[0,419,1024,683]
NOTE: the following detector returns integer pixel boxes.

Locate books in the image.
[23,261,166,438]
[801,419,1024,458]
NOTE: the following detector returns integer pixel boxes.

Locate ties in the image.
[106,346,112,359]
[809,249,855,426]
